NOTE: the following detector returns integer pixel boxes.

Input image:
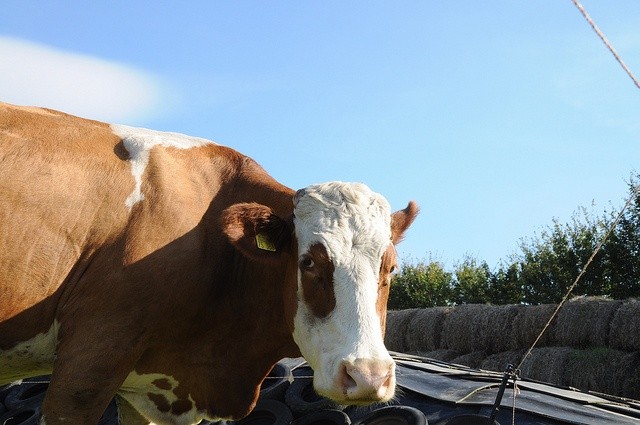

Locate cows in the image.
[0,100,422,425]
